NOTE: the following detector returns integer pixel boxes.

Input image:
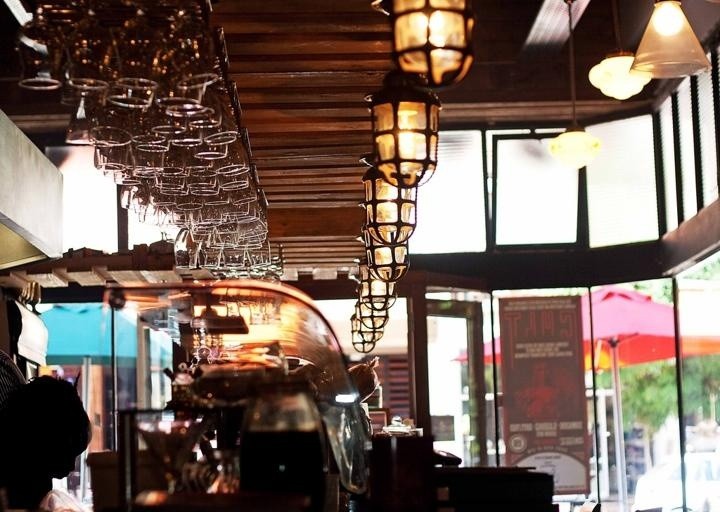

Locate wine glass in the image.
[12,0,277,282]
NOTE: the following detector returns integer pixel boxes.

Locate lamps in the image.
[362,66,445,189]
[587,2,659,103]
[628,0,712,79]
[368,1,480,94]
[345,151,418,353]
[543,0,605,174]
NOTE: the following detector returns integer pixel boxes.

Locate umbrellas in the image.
[452,288,719,505]
[34,302,173,510]
